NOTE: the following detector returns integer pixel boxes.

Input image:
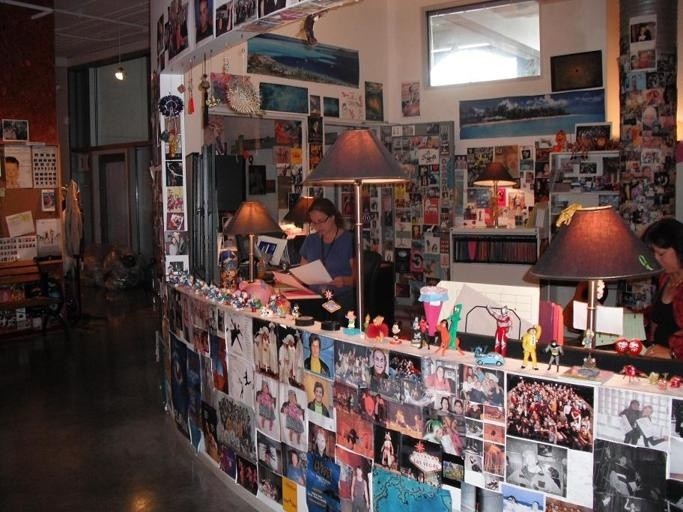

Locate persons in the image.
[485,306,511,356]
[521,325,540,371]
[259,439,282,505]
[278,389,306,444]
[507,377,592,447]
[255,381,276,432]
[339,311,359,336]
[379,433,395,467]
[216,8,229,31]
[350,467,368,511]
[620,401,668,448]
[300,200,359,321]
[641,219,683,364]
[522,454,541,475]
[2,156,20,189]
[619,16,679,123]
[437,305,463,357]
[168,234,177,254]
[256,326,304,386]
[390,355,505,480]
[307,429,339,498]
[283,451,302,482]
[544,341,563,372]
[197,0,211,37]
[305,335,328,376]
[603,449,662,507]
[310,382,329,416]
[334,346,394,427]
[344,429,360,449]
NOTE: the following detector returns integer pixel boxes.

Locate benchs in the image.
[0,261,72,337]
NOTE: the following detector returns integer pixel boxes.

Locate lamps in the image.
[301,126,413,333]
[114,67,127,82]
[525,203,664,368]
[222,200,282,284]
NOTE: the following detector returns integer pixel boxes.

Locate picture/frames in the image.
[2,119,29,143]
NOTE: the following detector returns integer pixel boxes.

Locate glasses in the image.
[311,216,329,225]
